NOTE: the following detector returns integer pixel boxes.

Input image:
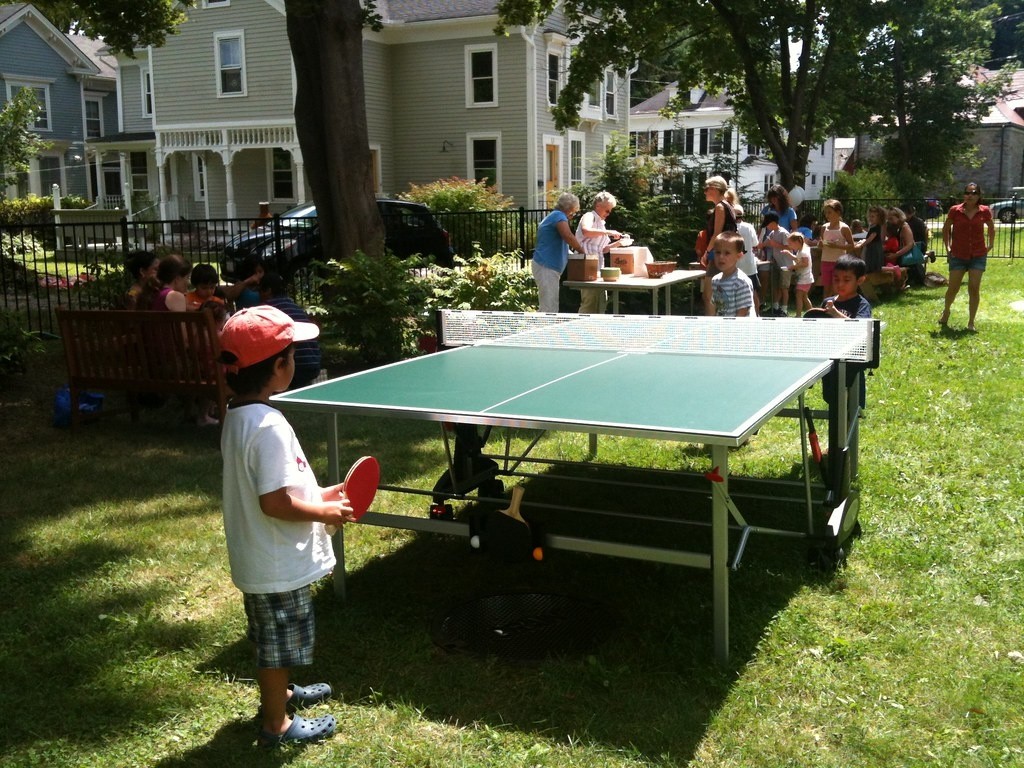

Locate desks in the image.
[810,239,865,286]
[268,321,889,659]
[562,269,707,316]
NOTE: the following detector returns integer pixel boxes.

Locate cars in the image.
[925,199,944,219]
[990,200,1024,223]
[220,197,454,284]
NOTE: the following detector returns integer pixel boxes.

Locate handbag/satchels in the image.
[899,239,926,266]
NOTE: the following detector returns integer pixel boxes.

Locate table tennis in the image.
[533,547,544,560]
[470,535,480,549]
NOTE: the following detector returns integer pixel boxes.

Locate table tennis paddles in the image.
[802,300,833,318]
[482,486,533,565]
[323,454,381,538]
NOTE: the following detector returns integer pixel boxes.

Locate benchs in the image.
[860,250,935,303]
[55,305,231,433]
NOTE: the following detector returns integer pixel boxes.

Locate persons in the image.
[531,192,586,313]
[124,250,320,427]
[574,192,622,313]
[820,255,872,407]
[938,182,995,332]
[220,306,354,746]
[695,176,936,316]
[709,230,760,444]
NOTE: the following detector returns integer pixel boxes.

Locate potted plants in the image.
[643,230,678,279]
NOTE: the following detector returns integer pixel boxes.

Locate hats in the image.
[760,212,779,228]
[733,205,743,216]
[218,304,321,373]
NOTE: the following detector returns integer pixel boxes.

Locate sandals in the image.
[257,682,330,718]
[260,712,336,748]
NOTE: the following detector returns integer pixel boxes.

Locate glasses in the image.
[963,190,978,196]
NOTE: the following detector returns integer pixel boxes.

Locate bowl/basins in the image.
[618,239,634,247]
[646,263,668,279]
[656,261,677,274]
[600,267,621,282]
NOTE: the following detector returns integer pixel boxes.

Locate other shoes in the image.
[939,311,950,324]
[767,307,788,317]
[968,324,975,332]
[930,250,936,263]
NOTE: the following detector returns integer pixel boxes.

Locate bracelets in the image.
[704,249,710,255]
[603,230,607,237]
[578,245,582,252]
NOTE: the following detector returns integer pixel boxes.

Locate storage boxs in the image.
[611,247,648,276]
[566,253,599,282]
